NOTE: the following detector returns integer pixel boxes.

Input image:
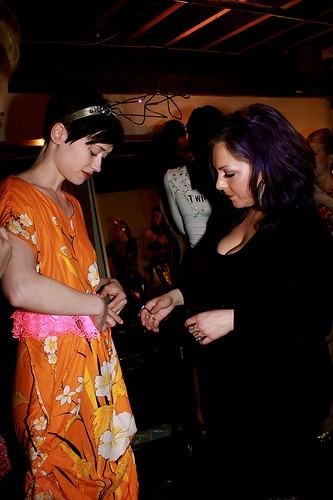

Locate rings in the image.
[141,305,150,313]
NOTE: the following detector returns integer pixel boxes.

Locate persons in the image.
[105,217,141,298]
[150,120,192,171]
[163,105,237,245]
[303,127,333,213]
[140,102,333,500]
[141,205,178,284]
[0,86,138,499]
